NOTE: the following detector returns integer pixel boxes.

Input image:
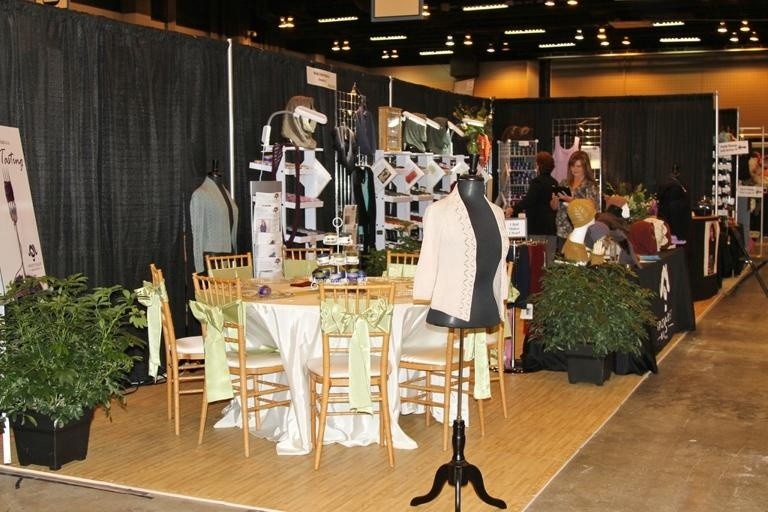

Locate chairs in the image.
[482,257,514,423]
[148,266,206,433]
[204,255,258,282]
[400,307,486,446]
[307,281,397,470]
[385,248,427,282]
[281,247,334,284]
[191,275,288,460]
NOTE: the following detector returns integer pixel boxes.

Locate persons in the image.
[505,152,559,270]
[412,173,511,330]
[190,172,239,290]
[654,163,688,237]
[560,199,605,266]
[606,195,631,220]
[550,151,599,260]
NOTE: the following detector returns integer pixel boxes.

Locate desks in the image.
[225,280,436,440]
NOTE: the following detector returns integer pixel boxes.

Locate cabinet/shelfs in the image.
[253,105,325,244]
[498,123,540,208]
[334,108,493,241]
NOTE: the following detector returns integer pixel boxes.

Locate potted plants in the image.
[1,271,152,472]
[519,253,661,386]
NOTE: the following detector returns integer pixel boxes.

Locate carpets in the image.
[2,257,767,512]
